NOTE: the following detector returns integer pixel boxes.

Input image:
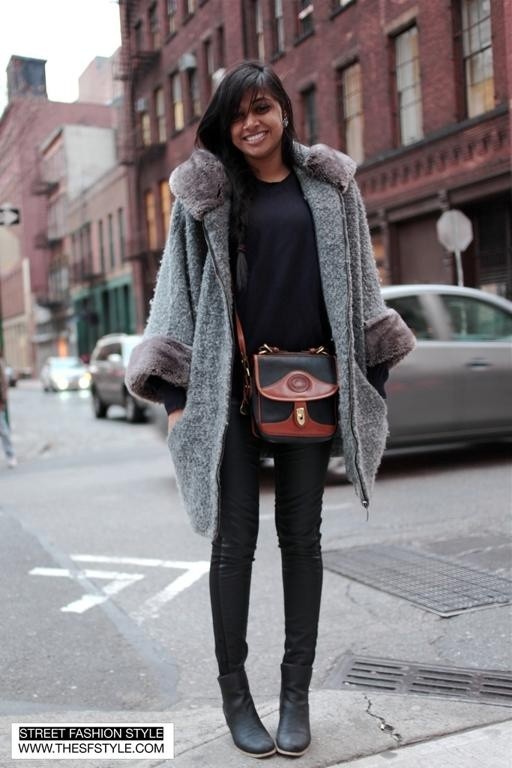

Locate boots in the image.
[217,669,277,759]
[275,662,313,757]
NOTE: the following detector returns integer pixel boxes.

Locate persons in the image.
[123,58,419,758]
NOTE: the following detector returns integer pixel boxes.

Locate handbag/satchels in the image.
[231,344,339,448]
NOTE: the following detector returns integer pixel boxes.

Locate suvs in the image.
[87,328,147,425]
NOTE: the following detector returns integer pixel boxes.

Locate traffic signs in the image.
[0,206,22,228]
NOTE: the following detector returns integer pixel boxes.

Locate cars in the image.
[14,362,33,380]
[2,360,20,387]
[261,285,512,483]
[37,354,93,394]
